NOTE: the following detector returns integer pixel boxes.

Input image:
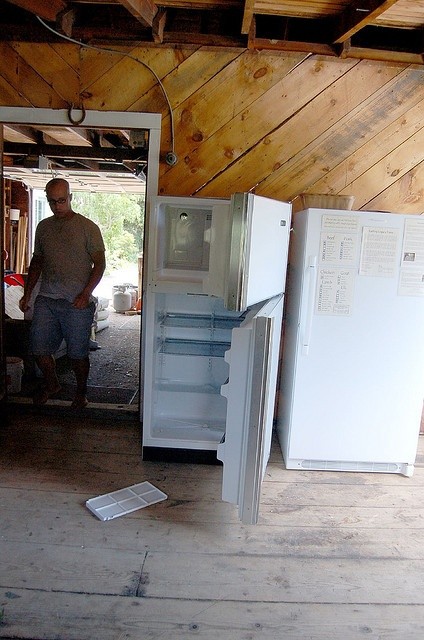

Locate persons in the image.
[17,178,107,409]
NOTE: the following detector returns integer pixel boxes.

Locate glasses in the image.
[49,197,66,205]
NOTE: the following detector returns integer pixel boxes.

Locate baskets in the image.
[300,193,356,210]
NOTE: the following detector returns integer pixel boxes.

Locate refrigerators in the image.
[276,205,424,480]
[140,192,293,528]
